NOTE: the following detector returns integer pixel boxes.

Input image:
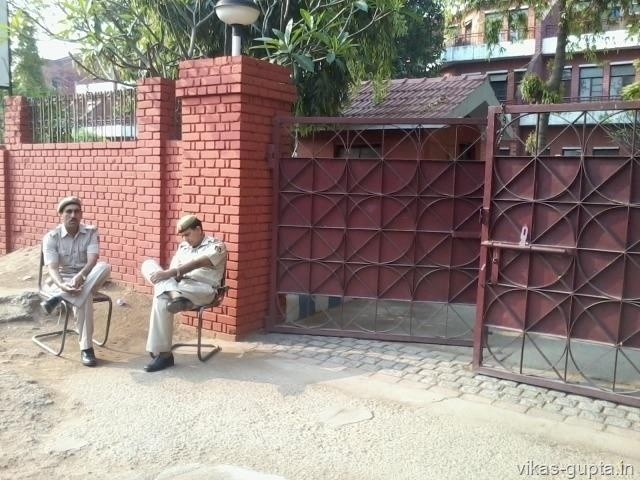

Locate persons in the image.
[140,214,229,372]
[40,195,112,366]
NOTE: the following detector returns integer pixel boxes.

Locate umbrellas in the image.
[81,348,97,367]
[40,297,59,317]
[166,297,193,314]
[143,352,174,372]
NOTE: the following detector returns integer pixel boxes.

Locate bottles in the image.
[177,214,196,234]
[57,196,82,214]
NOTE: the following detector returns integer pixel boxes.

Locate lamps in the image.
[149,258,230,363]
[32,245,113,356]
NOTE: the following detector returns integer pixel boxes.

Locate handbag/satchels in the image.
[174,267,182,279]
[79,273,87,281]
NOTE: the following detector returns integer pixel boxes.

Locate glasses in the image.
[213,1,262,56]
